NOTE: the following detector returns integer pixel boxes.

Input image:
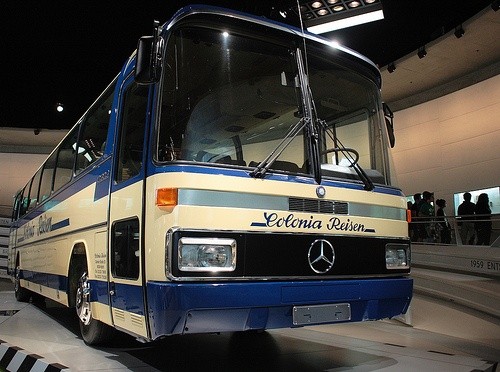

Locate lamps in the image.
[57,99,64,112]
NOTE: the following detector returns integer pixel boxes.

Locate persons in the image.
[458,193,475,245]
[417,191,436,243]
[410,193,422,241]
[475,193,492,246]
[408,201,413,235]
[436,199,450,242]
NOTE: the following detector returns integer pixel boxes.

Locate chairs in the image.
[192,150,230,164]
[268,161,299,171]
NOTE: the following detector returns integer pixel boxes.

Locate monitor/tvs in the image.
[454,186,500,219]
[405,194,434,206]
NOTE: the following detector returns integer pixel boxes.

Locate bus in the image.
[6,4,414,348]
